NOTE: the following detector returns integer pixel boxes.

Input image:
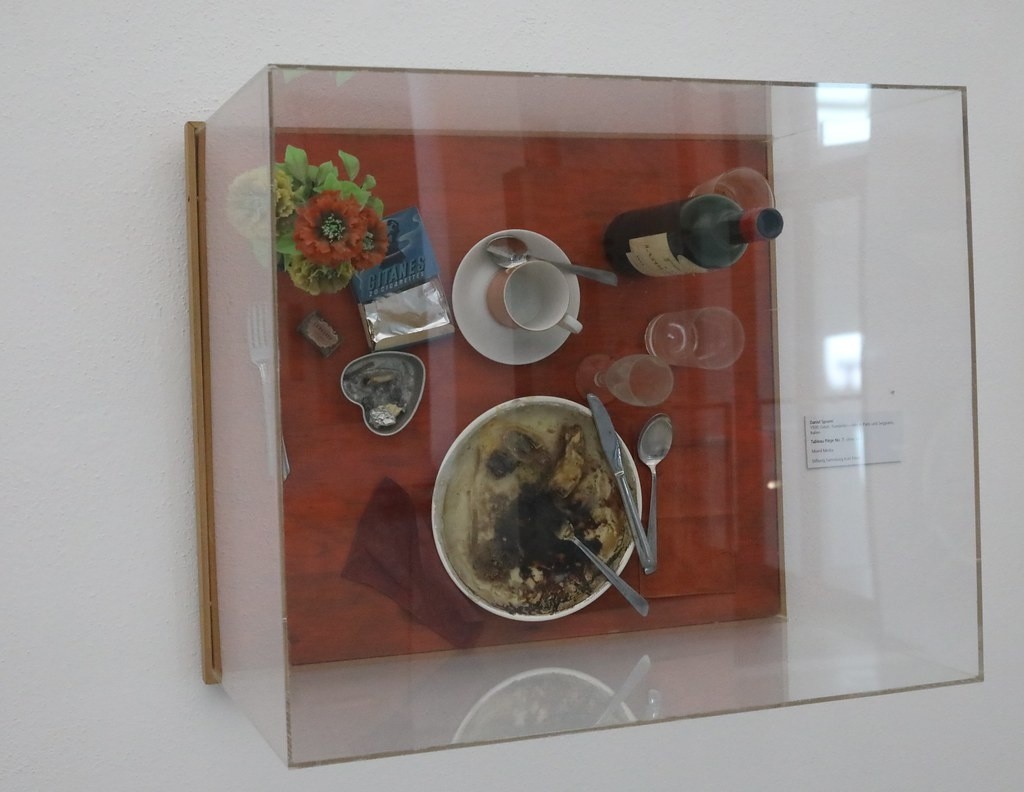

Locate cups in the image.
[486,261,583,334]
[687,166,775,211]
[644,306,745,370]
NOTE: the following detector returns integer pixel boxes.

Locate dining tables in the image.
[208,126,782,666]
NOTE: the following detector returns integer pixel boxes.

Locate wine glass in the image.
[573,353,674,407]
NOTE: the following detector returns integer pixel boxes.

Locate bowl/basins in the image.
[339,350,427,437]
[452,667,639,746]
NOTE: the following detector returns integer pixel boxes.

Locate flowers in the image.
[226,145,389,295]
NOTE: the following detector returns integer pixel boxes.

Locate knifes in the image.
[586,391,655,571]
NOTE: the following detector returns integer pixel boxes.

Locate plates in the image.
[452,229,581,365]
[430,395,642,622]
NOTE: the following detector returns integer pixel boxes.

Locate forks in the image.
[552,518,649,617]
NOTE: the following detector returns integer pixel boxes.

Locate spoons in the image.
[637,412,673,575]
[487,235,618,287]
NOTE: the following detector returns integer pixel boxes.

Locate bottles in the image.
[604,194,784,279]
